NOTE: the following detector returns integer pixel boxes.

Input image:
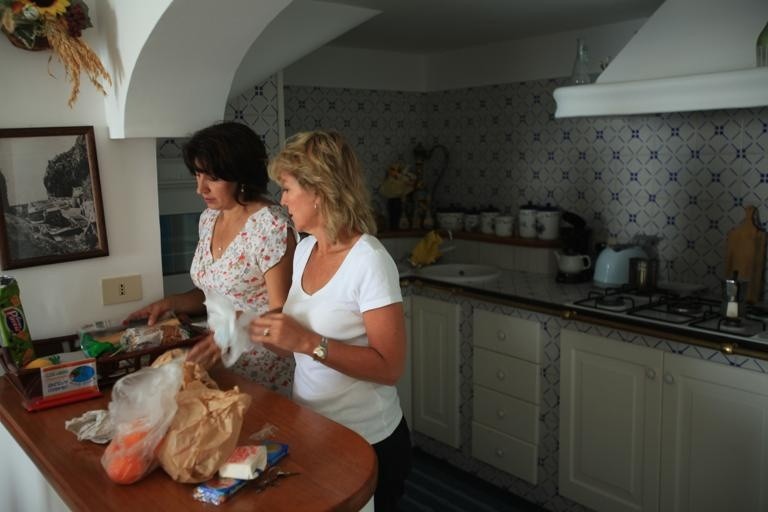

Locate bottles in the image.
[569,34,591,85]
[398,194,434,231]
[436,199,560,241]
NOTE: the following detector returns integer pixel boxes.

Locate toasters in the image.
[592,244,650,289]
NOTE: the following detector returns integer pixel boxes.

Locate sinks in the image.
[420,263,504,291]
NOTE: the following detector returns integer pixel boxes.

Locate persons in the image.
[122,118,297,400]
[242,125,411,510]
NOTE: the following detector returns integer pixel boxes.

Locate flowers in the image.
[1,0,91,27]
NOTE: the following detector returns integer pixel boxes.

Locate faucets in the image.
[405,226,453,267]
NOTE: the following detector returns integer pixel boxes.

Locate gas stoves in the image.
[563,282,767,350]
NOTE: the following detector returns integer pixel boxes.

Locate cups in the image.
[628,256,659,293]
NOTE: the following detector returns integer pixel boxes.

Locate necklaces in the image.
[216,220,239,250]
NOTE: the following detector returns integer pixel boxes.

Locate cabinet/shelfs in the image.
[412,290,460,454]
[555,324,768,511]
[472,304,540,488]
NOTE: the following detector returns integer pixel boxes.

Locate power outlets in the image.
[101,275,142,305]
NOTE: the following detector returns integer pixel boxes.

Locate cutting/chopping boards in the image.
[722,204,767,310]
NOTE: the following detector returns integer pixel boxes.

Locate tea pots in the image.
[718,269,747,319]
[552,250,591,274]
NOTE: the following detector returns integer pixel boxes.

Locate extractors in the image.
[551,0,767,120]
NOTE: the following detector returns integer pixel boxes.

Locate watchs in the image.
[310,336,328,364]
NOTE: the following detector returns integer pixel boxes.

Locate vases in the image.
[2,19,82,51]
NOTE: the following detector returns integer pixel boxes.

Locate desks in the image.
[1,350,381,511]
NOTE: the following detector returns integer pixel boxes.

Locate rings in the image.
[263,327,270,336]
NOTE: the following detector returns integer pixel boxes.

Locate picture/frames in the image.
[0,125,111,274]
